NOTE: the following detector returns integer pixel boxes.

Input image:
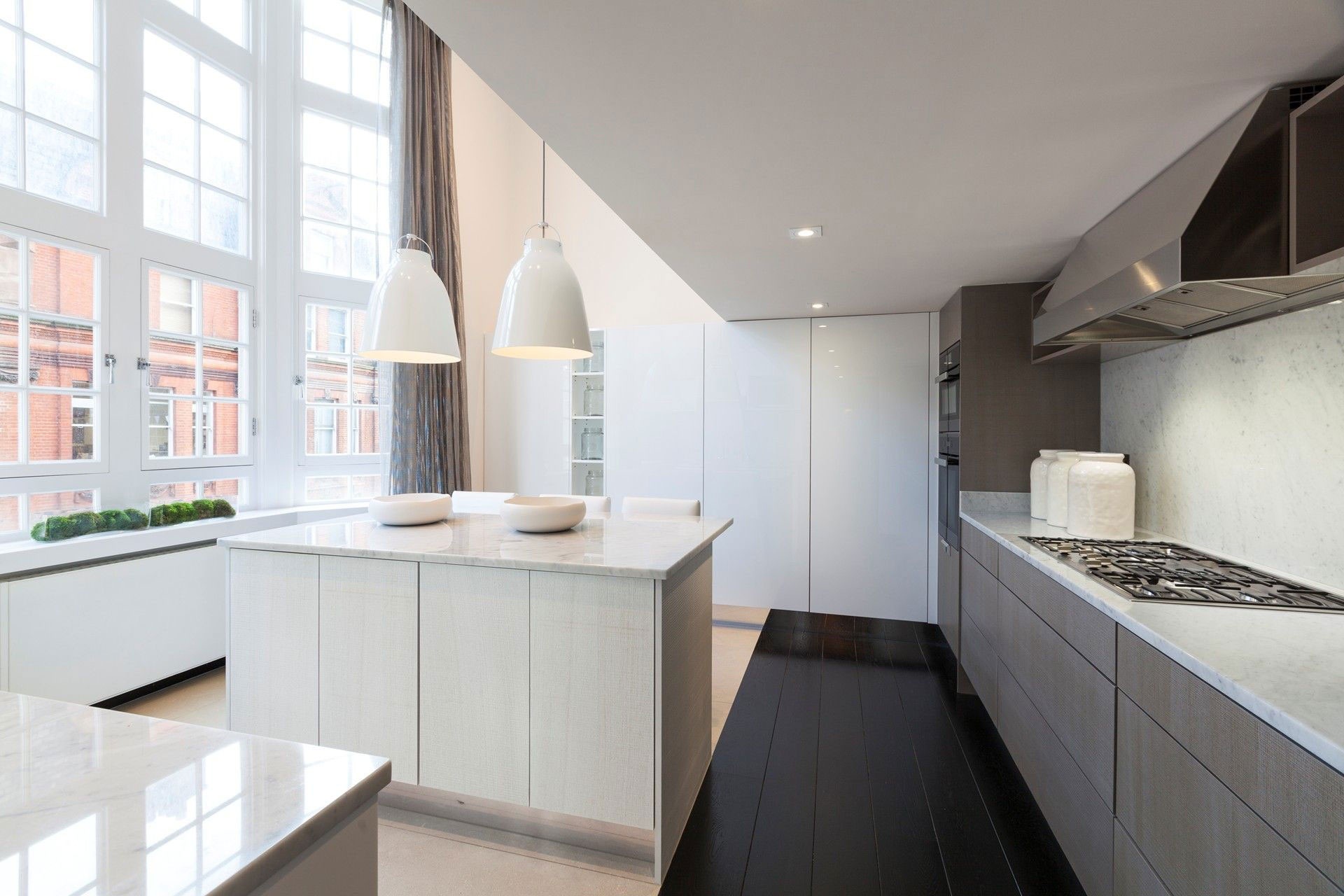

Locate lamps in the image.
[488,139,594,362]
[358,232,462,365]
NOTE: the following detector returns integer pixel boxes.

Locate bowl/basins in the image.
[503,496,586,532]
[369,493,452,525]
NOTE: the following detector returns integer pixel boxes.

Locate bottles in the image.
[586,470,603,496]
[583,385,604,416]
[1031,449,1076,519]
[1046,452,1100,528]
[1067,451,1136,540]
[583,341,603,372]
[581,427,604,460]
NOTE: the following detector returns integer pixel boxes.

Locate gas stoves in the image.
[1015,537,1344,611]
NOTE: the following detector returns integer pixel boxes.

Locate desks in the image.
[222,501,735,889]
[0,689,395,896]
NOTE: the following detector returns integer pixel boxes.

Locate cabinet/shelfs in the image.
[227,546,419,789]
[570,330,604,496]
[416,563,657,834]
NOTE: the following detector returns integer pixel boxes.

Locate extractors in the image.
[1032,85,1289,355]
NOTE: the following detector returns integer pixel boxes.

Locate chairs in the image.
[451,490,517,507]
[621,496,701,517]
[541,493,610,514]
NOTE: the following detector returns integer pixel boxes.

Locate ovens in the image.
[935,339,959,551]
